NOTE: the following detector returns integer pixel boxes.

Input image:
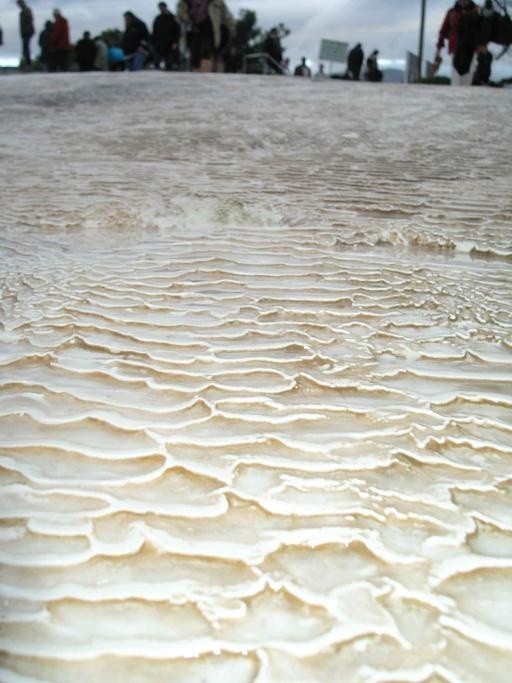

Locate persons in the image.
[436,0,485,85]
[294,57,311,76]
[38,0,283,72]
[348,43,364,80]
[368,50,379,79]
[16,0,34,64]
[313,63,327,77]
